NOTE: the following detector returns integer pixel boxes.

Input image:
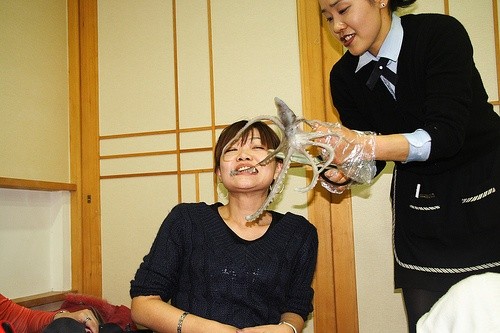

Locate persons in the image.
[128,120,320,333]
[0,294,101,333]
[306,0,500,333]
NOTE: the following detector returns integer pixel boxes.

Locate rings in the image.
[82,317,91,324]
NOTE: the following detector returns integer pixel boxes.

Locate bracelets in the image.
[176,311,191,333]
[279,320,298,333]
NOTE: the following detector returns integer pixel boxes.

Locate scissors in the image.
[268,148,353,186]
